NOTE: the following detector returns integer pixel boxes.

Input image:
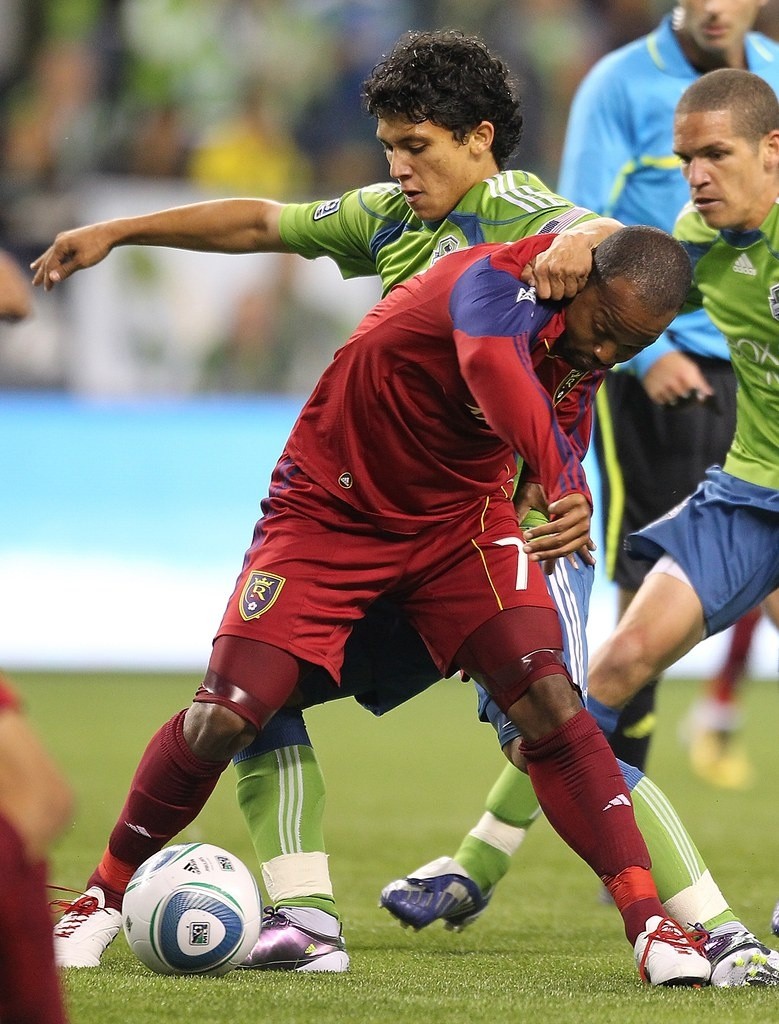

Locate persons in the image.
[30,34,779,990]
[552,0,779,778]
[380,66,779,935]
[35,224,716,994]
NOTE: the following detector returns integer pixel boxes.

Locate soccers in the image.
[121,843,261,979]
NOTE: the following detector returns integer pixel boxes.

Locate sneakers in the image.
[377,856,496,935]
[47,885,123,968]
[233,905,349,973]
[632,915,779,987]
